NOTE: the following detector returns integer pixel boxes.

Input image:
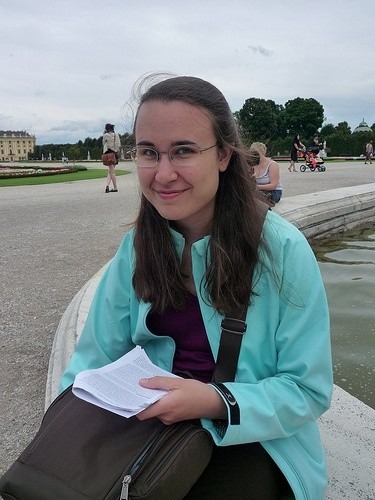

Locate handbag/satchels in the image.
[1,382,214,500]
[101,152,118,167]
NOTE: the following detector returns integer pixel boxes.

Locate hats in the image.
[105,123,115,131]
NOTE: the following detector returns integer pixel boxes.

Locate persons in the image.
[364,139,374,165]
[58,73,333,500]
[62,156,69,163]
[103,123,121,193]
[249,141,282,211]
[288,134,323,172]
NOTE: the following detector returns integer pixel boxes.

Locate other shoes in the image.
[106,186,109,193]
[109,189,118,192]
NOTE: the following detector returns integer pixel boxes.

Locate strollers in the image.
[300,146,327,172]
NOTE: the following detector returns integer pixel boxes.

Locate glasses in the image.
[127,142,221,168]
